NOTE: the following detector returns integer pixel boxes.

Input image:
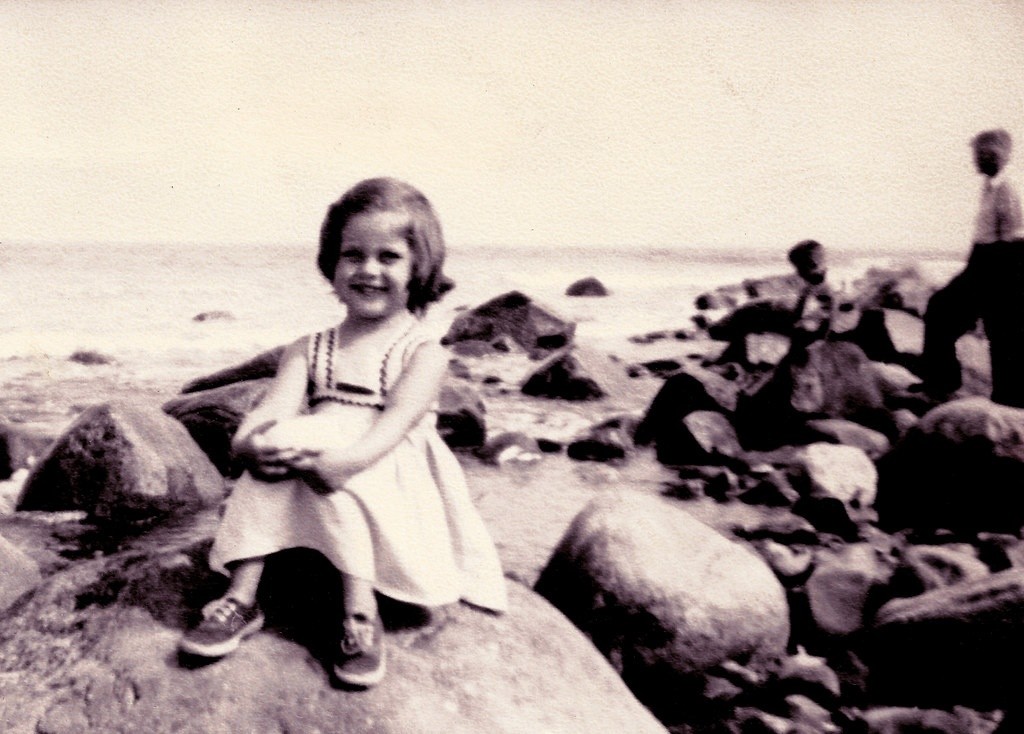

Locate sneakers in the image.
[334,610,389,686]
[179,596,264,657]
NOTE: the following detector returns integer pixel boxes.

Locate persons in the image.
[789,124,1024,408]
[180,174,510,688]
